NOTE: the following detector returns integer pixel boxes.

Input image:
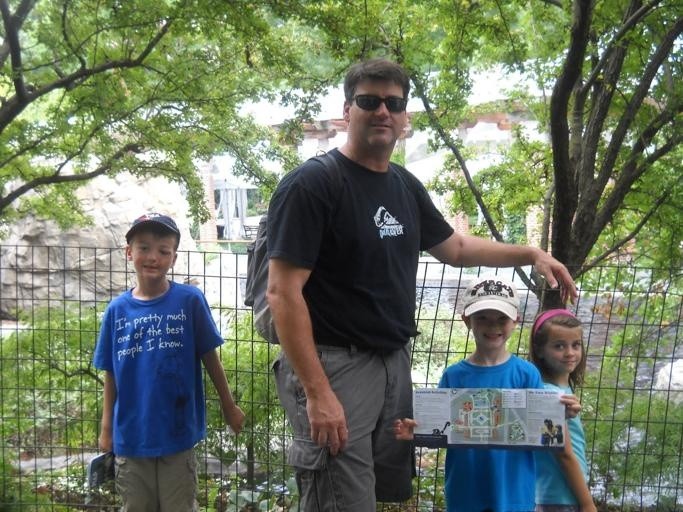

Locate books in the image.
[87,451,113,491]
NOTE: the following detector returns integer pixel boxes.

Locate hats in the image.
[124,211,180,252]
[460,274,521,319]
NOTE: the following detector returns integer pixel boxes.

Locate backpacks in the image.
[241,152,341,349]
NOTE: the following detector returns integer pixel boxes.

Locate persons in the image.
[91,212,246,511]
[270,58,579,511]
[526,308,599,512]
[540,419,564,445]
[393,274,582,512]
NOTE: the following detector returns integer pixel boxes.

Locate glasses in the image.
[349,92,408,114]
